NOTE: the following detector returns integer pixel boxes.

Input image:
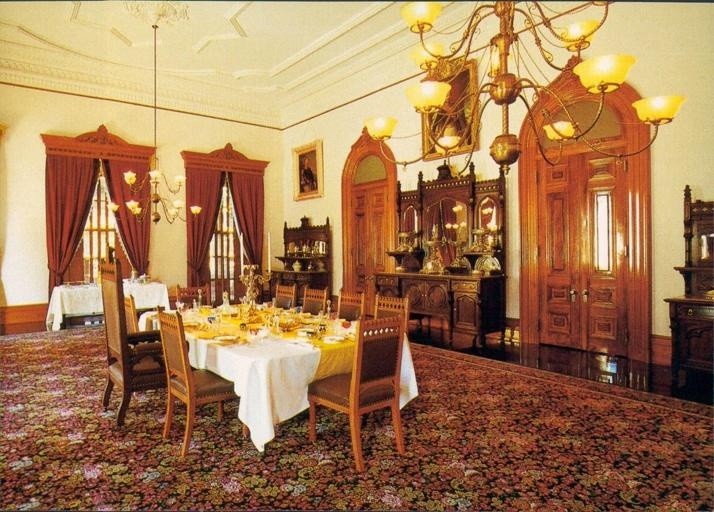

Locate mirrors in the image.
[386,158,506,272]
[672,182,713,299]
[286,237,329,258]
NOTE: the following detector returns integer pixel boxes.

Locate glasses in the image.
[360,2,689,180]
[101,22,206,228]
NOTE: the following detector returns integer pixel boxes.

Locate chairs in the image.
[156,305,250,458]
[307,310,405,471]
[100,258,190,428]
[125,245,411,437]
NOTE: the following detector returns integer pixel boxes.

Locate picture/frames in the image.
[42,277,171,334]
[418,56,481,163]
[287,138,325,204]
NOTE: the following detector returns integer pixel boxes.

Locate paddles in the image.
[0,309,710,510]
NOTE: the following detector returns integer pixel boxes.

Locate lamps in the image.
[360,2,689,180]
[101,22,206,228]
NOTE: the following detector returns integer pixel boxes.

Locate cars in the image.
[174,288,350,348]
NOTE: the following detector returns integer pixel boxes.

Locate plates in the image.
[662,293,713,398]
[369,267,508,357]
[264,213,333,310]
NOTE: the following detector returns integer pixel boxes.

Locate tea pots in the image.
[386,158,506,272]
[672,182,713,299]
[286,237,329,258]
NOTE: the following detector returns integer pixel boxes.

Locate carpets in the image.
[0,309,710,510]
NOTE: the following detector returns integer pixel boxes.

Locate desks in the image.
[42,277,171,334]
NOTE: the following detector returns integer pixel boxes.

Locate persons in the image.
[300,158,316,191]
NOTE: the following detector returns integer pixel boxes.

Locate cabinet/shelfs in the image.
[264,213,333,310]
[662,293,713,398]
[369,267,508,357]
[130,269,152,285]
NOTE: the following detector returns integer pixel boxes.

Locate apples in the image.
[265,230,272,274]
[238,231,247,276]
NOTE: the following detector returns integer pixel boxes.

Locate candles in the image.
[238,231,247,276]
[265,230,272,274]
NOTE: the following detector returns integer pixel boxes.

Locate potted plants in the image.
[418,56,481,163]
[287,138,325,204]
